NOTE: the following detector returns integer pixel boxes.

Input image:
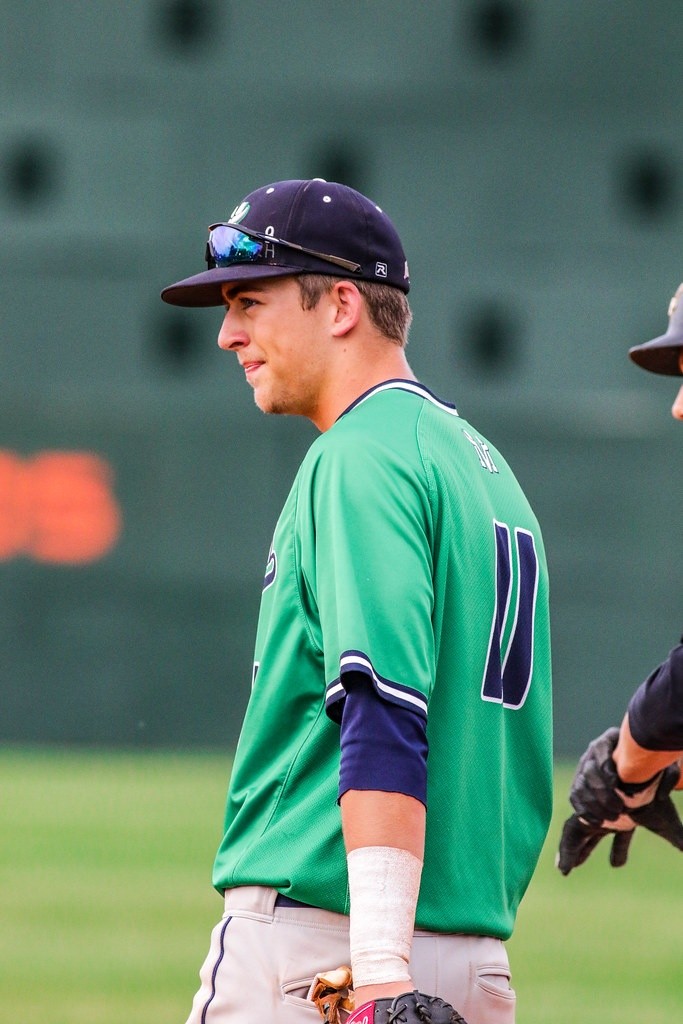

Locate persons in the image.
[162,177,552,1022]
[558,280,683,873]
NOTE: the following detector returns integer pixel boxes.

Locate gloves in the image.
[569,727,682,824]
[556,793,683,875]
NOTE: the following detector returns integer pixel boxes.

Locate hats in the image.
[628,281,683,377]
[161,175,413,308]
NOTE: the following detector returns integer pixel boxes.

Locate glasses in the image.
[204,222,364,275]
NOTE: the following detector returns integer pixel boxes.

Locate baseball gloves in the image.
[342,990,468,1024]
